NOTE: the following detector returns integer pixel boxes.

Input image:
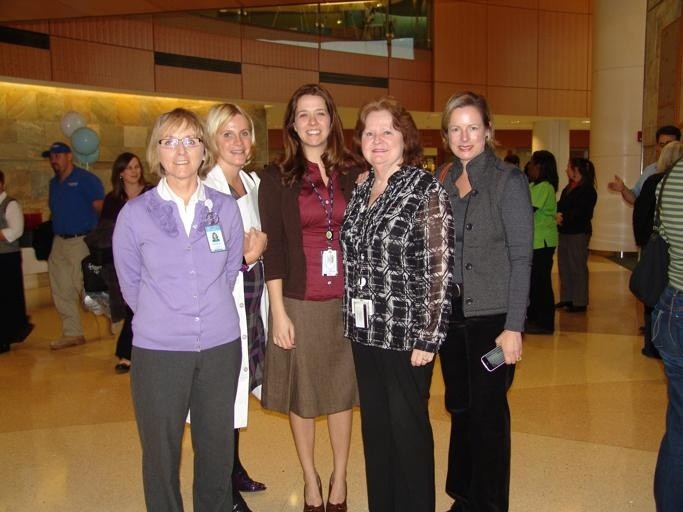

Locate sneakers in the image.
[51,337,85,347]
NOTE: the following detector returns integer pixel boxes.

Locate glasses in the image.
[157,138,204,148]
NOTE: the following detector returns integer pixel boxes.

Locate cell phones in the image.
[480,346,505,371]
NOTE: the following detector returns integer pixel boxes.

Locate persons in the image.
[111,107,245,512]
[434,92,535,511]
[633,158,683,512]
[185,104,269,512]
[337,98,454,511]
[259,85,365,511]
[30,142,117,350]
[631,141,683,357]
[0,171,33,353]
[503,150,522,169]
[83,152,156,374]
[608,131,681,357]
[526,151,559,334]
[555,158,596,311]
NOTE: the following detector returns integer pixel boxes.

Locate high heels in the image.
[303,473,325,512]
[325,472,348,512]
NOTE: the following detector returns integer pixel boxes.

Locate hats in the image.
[41,142,71,157]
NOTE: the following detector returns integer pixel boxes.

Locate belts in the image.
[56,232,86,239]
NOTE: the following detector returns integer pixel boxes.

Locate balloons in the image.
[59,111,86,137]
[70,129,99,153]
[71,146,102,164]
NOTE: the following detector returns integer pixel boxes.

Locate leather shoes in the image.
[114,362,130,374]
[554,300,567,309]
[232,492,252,512]
[238,470,268,493]
[566,305,588,312]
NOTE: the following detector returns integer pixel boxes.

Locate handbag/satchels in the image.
[629,156,672,307]
